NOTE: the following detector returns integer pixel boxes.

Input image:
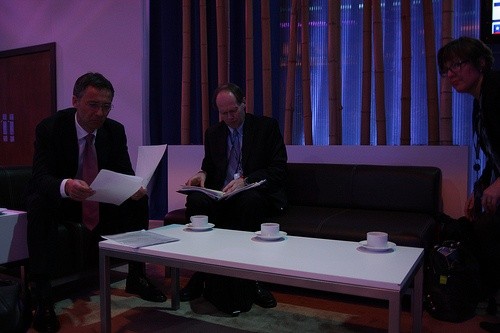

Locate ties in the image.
[81,133,99,231]
[224,129,240,190]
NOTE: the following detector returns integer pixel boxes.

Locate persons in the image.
[28,73,167,333]
[172,83,287,308]
[437,36,500,333]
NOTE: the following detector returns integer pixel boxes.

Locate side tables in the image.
[0,207,29,298]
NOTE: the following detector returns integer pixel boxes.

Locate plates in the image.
[254,231,287,240]
[186,223,215,230]
[359,240,397,251]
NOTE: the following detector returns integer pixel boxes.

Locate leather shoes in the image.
[251,278,277,308]
[33,300,60,333]
[180,273,211,300]
[125,277,166,303]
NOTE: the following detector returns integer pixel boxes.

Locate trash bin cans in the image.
[425,214,482,321]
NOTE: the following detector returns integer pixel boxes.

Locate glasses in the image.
[442,60,469,82]
[75,99,113,112]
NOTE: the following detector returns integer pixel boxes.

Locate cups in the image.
[260,222,280,237]
[190,215,209,228]
[366,231,388,248]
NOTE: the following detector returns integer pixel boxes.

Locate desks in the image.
[99,224,425,333]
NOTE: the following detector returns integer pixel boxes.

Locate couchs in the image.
[162,163,443,277]
[0,166,128,286]
